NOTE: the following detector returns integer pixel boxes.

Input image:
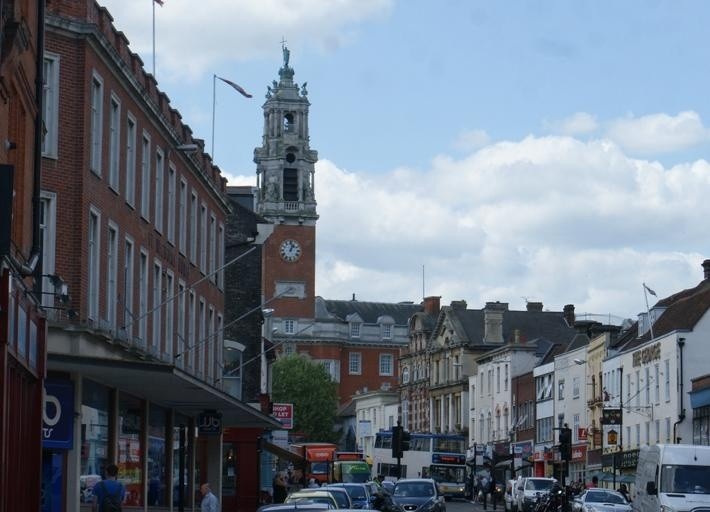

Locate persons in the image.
[466,474,522,512]
[282,45,290,65]
[91,464,125,512]
[617,482,635,508]
[364,474,393,512]
[272,467,322,504]
[584,475,598,489]
[199,480,219,512]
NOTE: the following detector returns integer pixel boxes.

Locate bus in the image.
[371,428,467,499]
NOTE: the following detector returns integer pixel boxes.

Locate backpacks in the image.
[100,481,122,512]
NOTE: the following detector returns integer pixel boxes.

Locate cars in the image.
[505,476,559,512]
[573,487,632,512]
[391,477,447,512]
[257,480,395,512]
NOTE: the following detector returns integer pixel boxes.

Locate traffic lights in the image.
[559,429,572,461]
[392,425,410,458]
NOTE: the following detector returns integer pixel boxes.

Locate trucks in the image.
[631,443,710,512]
[290,442,337,485]
[326,451,371,483]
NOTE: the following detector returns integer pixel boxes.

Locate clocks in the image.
[278,237,303,264]
